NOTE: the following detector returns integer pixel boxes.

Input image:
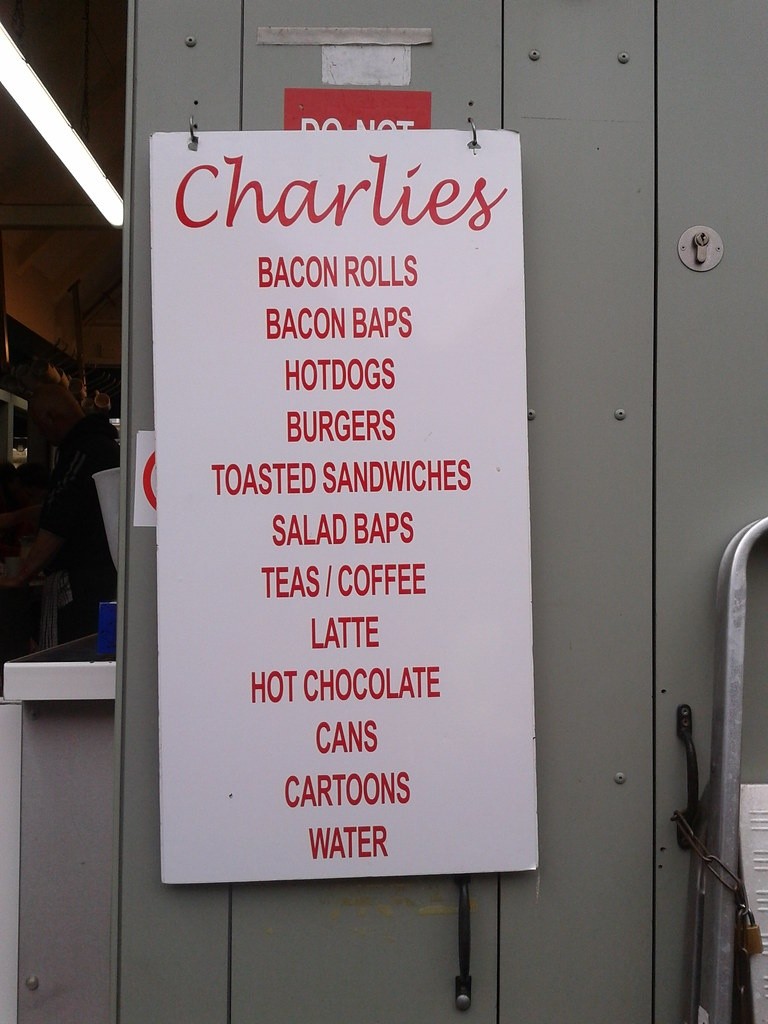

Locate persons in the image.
[0,383,117,654]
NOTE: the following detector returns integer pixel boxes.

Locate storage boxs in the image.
[98,600,116,656]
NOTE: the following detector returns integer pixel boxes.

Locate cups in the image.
[69,378,87,400]
[81,398,96,415]
[31,358,61,385]
[58,368,69,388]
[94,389,111,412]
